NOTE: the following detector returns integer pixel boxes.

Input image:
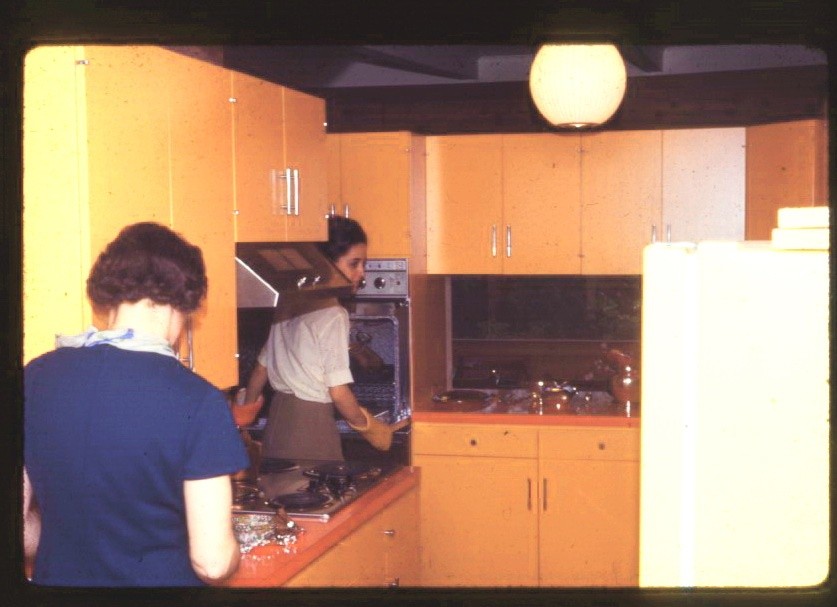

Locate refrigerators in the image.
[641,243,834,592]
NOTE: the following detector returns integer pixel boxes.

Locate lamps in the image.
[528,43,626,132]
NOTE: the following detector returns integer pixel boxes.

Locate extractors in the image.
[235,244,352,324]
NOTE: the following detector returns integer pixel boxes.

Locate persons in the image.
[231,213,393,461]
[24,221,250,588]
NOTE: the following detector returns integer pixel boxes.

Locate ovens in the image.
[242,257,411,467]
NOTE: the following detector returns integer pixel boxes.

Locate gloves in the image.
[231,387,263,426]
[345,404,392,451]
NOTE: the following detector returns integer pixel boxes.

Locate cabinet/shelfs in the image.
[744,119,825,240]
[581,126,746,277]
[283,487,421,587]
[411,423,638,587]
[90,47,240,394]
[427,136,581,276]
[232,70,329,244]
[328,132,412,269]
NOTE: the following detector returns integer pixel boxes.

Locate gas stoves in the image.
[222,452,401,523]
